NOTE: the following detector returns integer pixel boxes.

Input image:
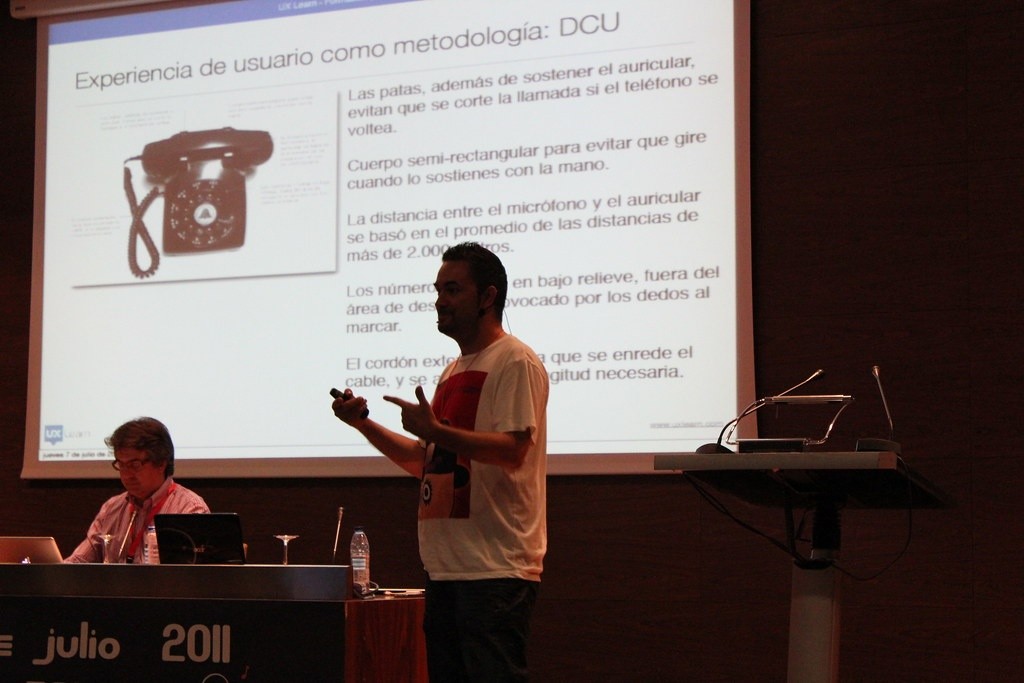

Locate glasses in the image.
[112,458,152,470]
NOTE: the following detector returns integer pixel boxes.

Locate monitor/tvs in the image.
[153,513,247,564]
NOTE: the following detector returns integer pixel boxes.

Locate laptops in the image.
[0,536,64,564]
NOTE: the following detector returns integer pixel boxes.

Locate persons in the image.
[62,417,212,565]
[331,242,550,683]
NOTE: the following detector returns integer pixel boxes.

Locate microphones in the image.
[852,366,902,461]
[118,511,139,563]
[331,506,344,565]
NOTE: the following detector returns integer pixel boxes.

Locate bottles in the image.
[142,525,160,564]
[349,525,370,595]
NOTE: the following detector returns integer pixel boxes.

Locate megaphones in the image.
[694,370,823,454]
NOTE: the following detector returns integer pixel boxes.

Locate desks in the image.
[0,564,430,683]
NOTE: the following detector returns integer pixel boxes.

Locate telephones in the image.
[142,126,274,252]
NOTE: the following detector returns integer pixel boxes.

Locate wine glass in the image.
[274,534,299,565]
[97,534,116,563]
